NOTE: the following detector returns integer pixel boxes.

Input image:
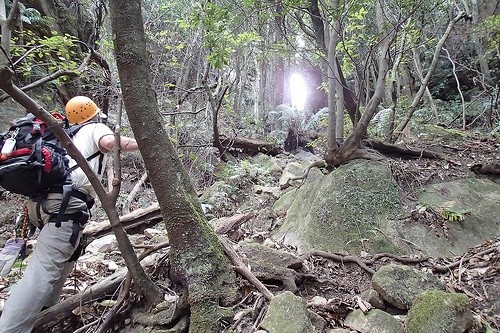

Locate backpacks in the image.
[0,111,73,197]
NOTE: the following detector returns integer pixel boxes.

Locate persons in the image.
[1,95,141,333]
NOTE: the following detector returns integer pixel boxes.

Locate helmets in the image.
[65,96,100,125]
